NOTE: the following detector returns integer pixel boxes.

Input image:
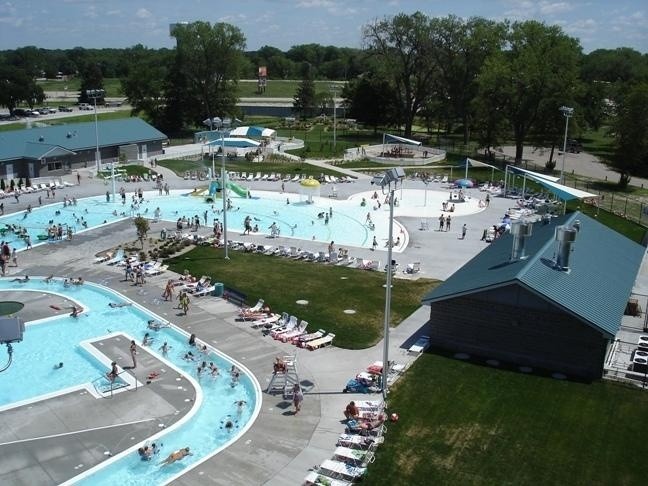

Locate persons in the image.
[0,115,597,485]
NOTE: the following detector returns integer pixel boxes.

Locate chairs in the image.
[0,178,77,200]
[116,169,357,185]
[96,247,336,352]
[266,349,301,396]
[303,350,408,486]
[178,230,419,274]
[416,171,553,245]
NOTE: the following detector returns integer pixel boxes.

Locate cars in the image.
[78,103,94,110]
[58,105,73,112]
[14,106,57,117]
[558,138,584,154]
[103,98,123,108]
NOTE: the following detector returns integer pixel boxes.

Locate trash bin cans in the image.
[215,282,224,297]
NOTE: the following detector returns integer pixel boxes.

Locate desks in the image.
[408,334,431,357]
